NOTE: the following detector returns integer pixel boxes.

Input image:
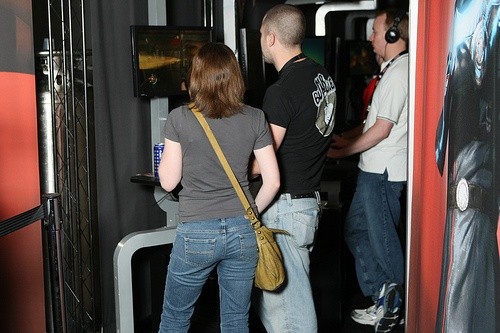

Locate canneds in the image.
[153,143,165,178]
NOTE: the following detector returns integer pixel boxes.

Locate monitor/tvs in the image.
[130,24,216,98]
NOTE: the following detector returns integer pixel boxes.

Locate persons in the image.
[157,41,280,333]
[250,3,339,332]
[328,10,409,332]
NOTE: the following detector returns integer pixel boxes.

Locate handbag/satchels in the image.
[251,218,290,292]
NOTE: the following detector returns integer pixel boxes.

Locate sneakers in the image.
[350,300,377,327]
[375,279,406,333]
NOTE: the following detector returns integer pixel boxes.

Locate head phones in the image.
[385,10,406,43]
